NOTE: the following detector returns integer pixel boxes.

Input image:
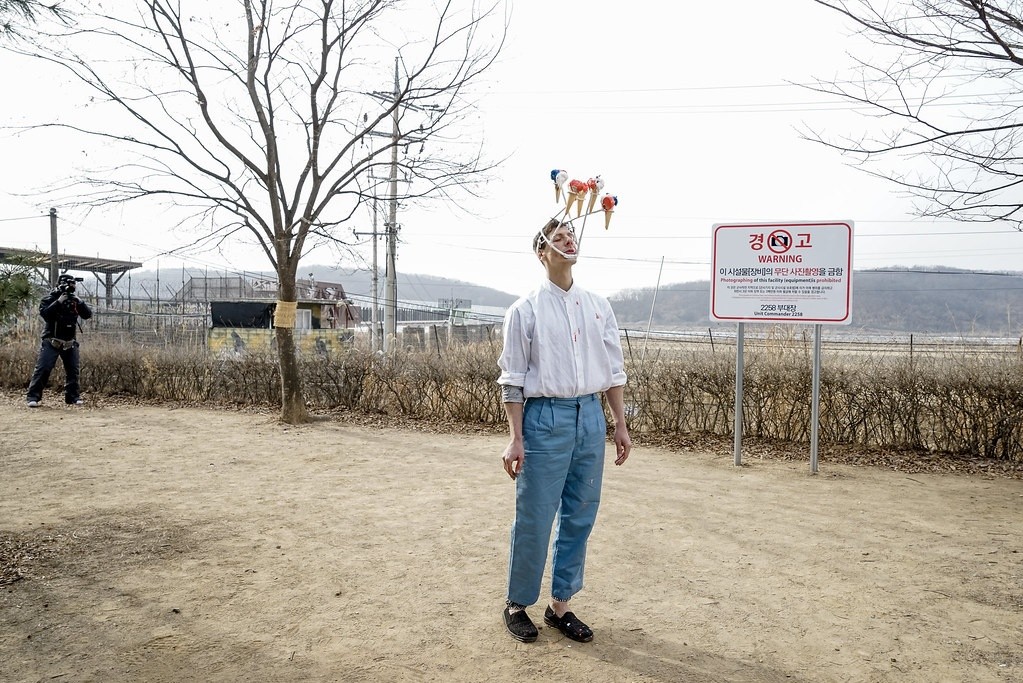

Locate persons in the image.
[497,218,632,643]
[26,275,93,407]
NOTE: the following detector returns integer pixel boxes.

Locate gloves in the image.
[58,292,68,304]
[70,292,82,304]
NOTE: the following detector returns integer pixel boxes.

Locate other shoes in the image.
[28,401,37,407]
[543,604,593,642]
[503,607,538,642]
[75,401,84,405]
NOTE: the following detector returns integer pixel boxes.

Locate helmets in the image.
[57,275,75,293]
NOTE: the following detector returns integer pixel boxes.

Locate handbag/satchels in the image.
[45,338,75,350]
